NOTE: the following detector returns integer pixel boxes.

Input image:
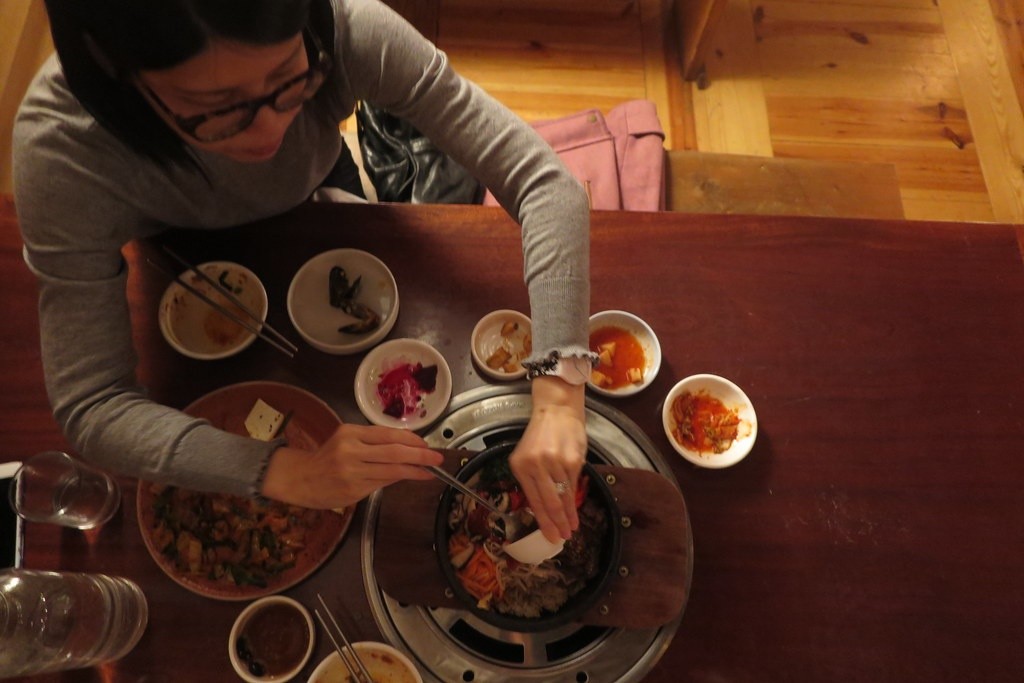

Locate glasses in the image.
[134,21,329,144]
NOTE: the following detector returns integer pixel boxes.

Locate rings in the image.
[555,479,571,497]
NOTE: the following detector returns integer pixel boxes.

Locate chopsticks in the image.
[419,465,499,516]
[315,593,374,683]
[147,248,297,360]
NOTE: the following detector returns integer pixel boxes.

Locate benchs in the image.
[666,150,905,220]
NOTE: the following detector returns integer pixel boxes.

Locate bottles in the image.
[0,571,147,678]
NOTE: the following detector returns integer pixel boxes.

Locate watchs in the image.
[526,355,597,384]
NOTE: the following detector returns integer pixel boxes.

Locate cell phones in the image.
[0,462,23,571]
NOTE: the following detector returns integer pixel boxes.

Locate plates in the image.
[287,249,398,354]
[136,381,355,600]
[353,338,452,429]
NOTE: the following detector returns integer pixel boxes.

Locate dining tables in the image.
[0,192,1024,683]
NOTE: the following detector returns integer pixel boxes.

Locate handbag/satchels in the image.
[355,94,488,205]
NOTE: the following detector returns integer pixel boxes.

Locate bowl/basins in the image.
[661,374,757,468]
[229,596,315,683]
[158,261,268,359]
[503,526,565,565]
[307,642,423,683]
[435,445,622,634]
[470,310,533,380]
[585,311,661,395]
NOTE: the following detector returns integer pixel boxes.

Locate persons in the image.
[14,0,593,546]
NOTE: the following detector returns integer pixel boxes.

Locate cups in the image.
[9,451,120,530]
